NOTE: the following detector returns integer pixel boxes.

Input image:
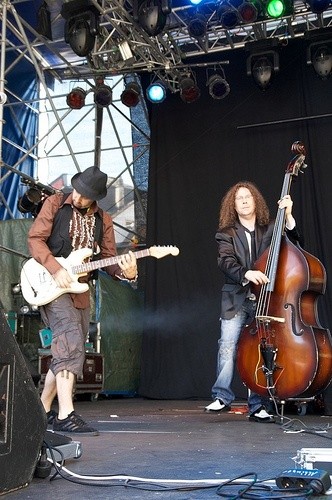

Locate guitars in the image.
[18,244,180,306]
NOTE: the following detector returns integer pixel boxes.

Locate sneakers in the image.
[46,410,58,429]
[53,410,98,436]
[249,406,276,423]
[204,398,231,414]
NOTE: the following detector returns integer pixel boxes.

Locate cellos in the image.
[233,141,332,423]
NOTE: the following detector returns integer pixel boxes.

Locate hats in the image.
[71,166,108,201]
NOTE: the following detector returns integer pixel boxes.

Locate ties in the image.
[244,227,257,293]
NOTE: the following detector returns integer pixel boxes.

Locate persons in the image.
[27,166,138,436]
[205,181,299,423]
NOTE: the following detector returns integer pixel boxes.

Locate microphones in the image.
[34,443,52,478]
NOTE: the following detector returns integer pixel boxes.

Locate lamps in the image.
[66,78,87,109]
[146,70,167,104]
[134,0,170,35]
[205,63,230,100]
[64,11,97,56]
[176,68,200,104]
[188,0,332,36]
[244,38,280,90]
[303,28,332,82]
[121,72,141,107]
[94,76,113,107]
[17,189,49,217]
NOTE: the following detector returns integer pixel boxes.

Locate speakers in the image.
[0,310,48,496]
